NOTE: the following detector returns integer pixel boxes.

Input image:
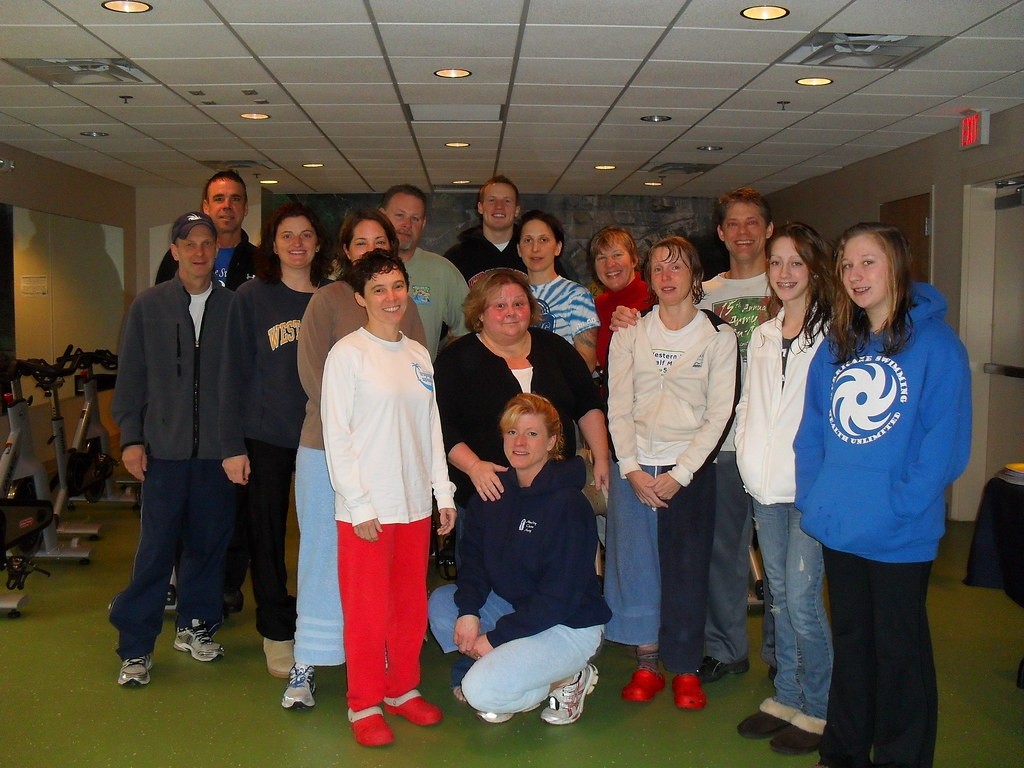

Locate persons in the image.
[111,171,776,710]
[428,392,612,725]
[320,247,456,745]
[732,221,834,756]
[791,222,970,768]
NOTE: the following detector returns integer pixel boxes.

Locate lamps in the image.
[650,176,676,211]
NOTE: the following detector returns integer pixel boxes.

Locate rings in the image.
[476,656,480,660]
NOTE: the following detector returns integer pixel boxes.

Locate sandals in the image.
[672,675,706,709]
[621,669,666,702]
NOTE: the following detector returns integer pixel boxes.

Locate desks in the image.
[962,477,1024,608]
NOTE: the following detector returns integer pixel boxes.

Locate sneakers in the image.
[118,653,153,686]
[281,662,317,709]
[540,663,598,725]
[173,619,224,662]
[768,666,777,689]
[701,656,749,683]
[476,702,540,723]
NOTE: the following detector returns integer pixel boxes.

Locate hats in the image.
[171,212,218,244]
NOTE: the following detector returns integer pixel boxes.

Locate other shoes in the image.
[263,637,296,679]
[769,711,828,755]
[737,697,802,739]
[383,689,443,726]
[165,584,178,610]
[348,706,392,746]
